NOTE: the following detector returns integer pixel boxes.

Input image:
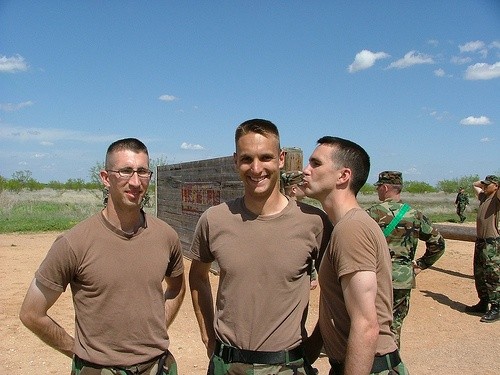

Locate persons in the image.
[302,136,410,375]
[470,175,500,318]
[281,170,318,291]
[101,184,109,211]
[364,171,445,352]
[188,119,334,375]
[140,192,151,210]
[19,139,186,375]
[455,186,470,223]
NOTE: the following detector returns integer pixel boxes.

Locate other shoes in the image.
[480,310,500,322]
[460,217,466,222]
[466,301,488,316]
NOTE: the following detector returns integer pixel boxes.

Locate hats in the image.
[480,175,500,184]
[372,171,403,186]
[280,171,303,188]
[459,186,464,190]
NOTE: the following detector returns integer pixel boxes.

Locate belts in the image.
[477,238,500,242]
[214,339,303,364]
[329,349,401,375]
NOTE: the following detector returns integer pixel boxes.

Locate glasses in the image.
[106,167,153,177]
